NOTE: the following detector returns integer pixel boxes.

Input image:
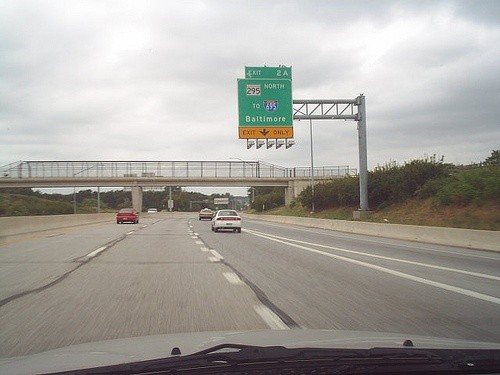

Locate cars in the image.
[198,208,217,220]
[147,208,158,213]
[116,207,140,224]
[211,210,242,233]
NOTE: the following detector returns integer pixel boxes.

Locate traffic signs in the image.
[238,78,294,139]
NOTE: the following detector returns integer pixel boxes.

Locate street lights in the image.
[229,157,245,177]
[73,164,103,214]
